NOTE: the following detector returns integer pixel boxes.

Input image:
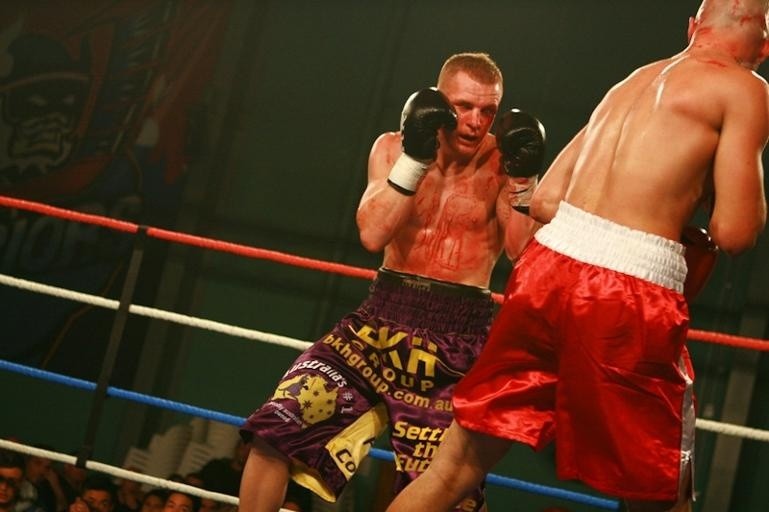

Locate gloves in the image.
[385,87,459,197]
[493,105,550,183]
[680,226,720,303]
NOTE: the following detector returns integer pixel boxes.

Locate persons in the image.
[453,493,482,512]
[238,53,547,512]
[379,0,768,512]
